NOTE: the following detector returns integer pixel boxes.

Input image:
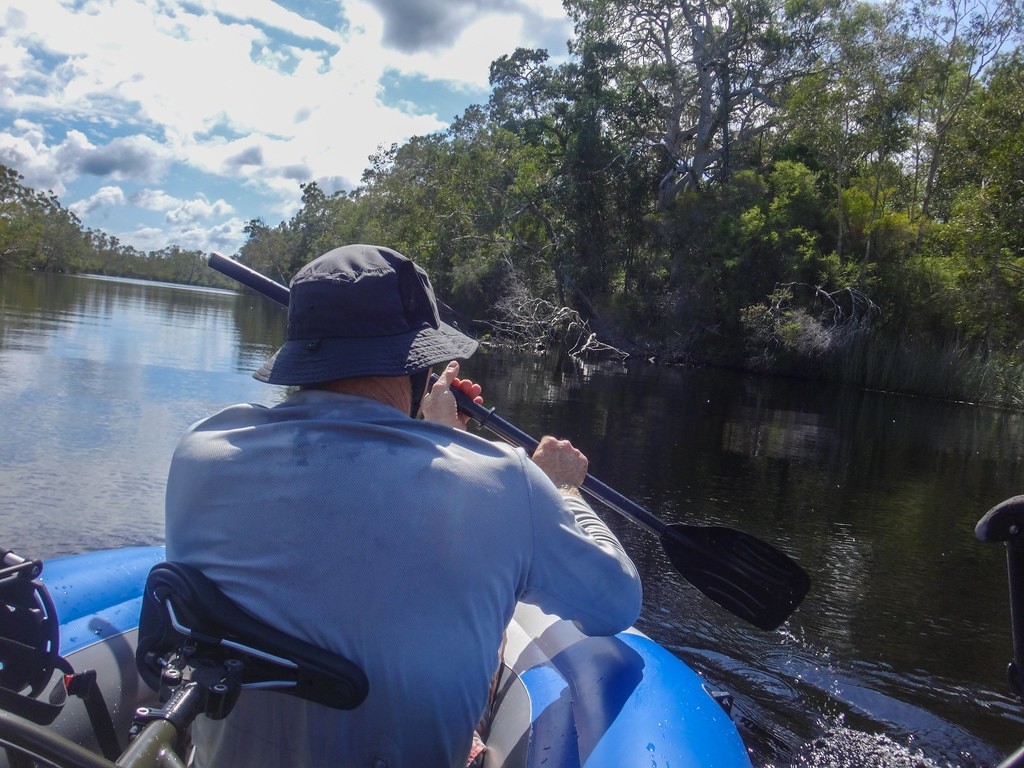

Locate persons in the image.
[165,243,644,768]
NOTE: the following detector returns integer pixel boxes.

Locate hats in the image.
[250,245,480,386]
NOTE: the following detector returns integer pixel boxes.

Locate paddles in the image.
[205,252,813,630]
